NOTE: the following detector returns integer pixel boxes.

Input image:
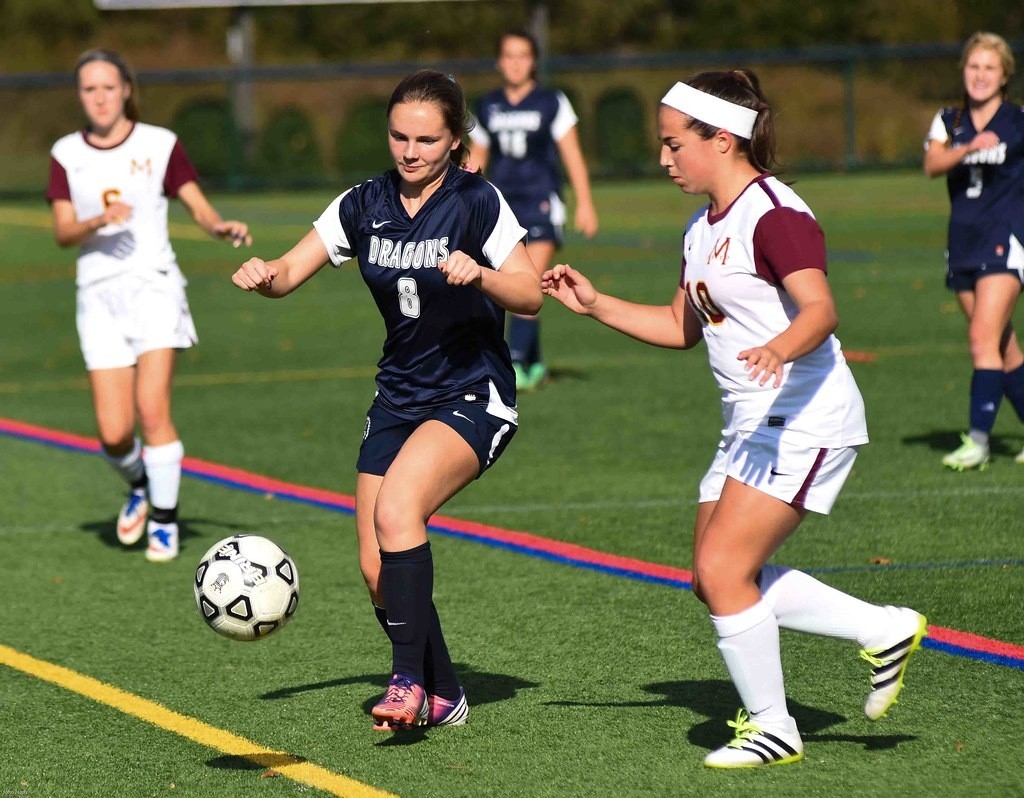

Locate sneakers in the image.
[704,706,806,770]
[856,604,928,721]
[940,430,992,473]
[373,687,470,732]
[1013,445,1024,463]
[116,487,148,546]
[371,673,429,727]
[143,519,181,562]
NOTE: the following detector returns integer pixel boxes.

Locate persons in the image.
[924,32,1024,472]
[540,70,930,769]
[230,70,544,732]
[462,29,599,392]
[45,47,252,563]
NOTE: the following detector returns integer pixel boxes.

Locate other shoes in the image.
[513,365,550,389]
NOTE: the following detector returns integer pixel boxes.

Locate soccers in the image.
[193,534,301,641]
